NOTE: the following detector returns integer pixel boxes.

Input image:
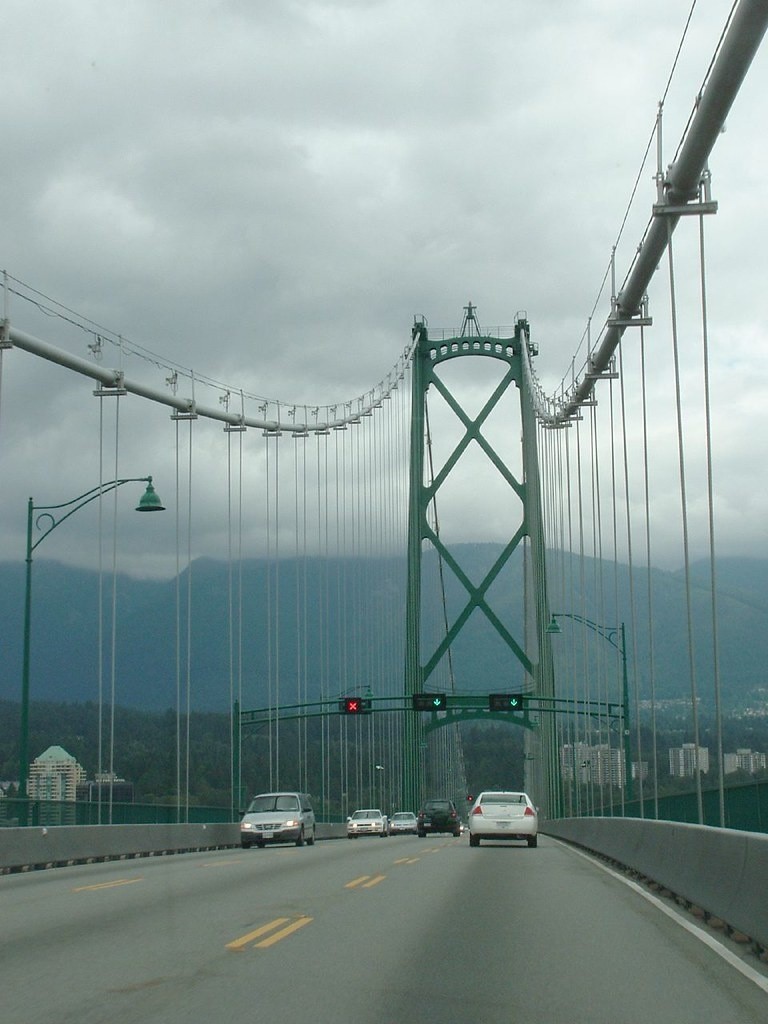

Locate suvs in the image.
[417,798,461,838]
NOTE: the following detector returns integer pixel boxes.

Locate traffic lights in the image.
[344,697,361,714]
[489,693,523,711]
[412,692,447,712]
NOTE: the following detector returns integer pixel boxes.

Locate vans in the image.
[238,791,317,849]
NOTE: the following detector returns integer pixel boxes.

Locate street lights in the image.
[318,684,374,823]
[544,612,635,818]
[18,473,168,827]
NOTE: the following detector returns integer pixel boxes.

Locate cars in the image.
[346,808,388,839]
[467,790,540,848]
[388,812,418,836]
